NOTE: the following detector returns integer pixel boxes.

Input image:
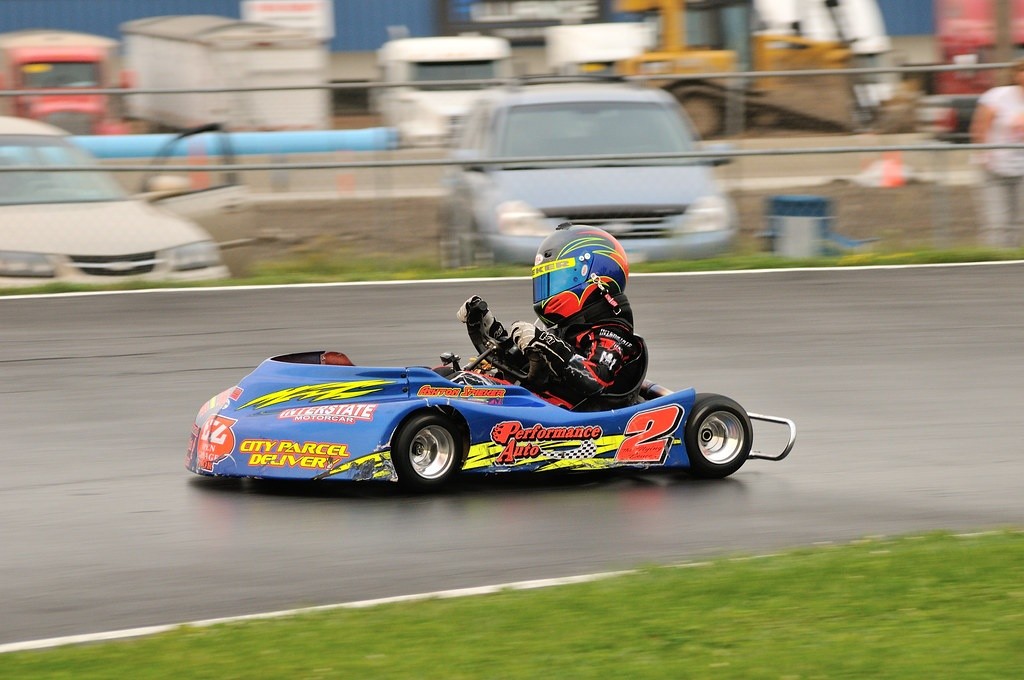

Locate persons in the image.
[452,219,653,419]
[863,70,927,138]
[957,52,1023,257]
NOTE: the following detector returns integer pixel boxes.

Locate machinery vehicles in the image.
[576,1,878,137]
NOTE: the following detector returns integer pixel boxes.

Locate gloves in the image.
[508,319,578,372]
[455,294,508,344]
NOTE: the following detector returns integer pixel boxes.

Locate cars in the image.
[1,110,248,296]
[455,84,746,269]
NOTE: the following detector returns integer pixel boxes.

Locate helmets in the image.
[532,220,628,331]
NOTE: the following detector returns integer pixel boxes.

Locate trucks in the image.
[365,34,514,152]
[0,27,139,139]
[914,0,1024,153]
